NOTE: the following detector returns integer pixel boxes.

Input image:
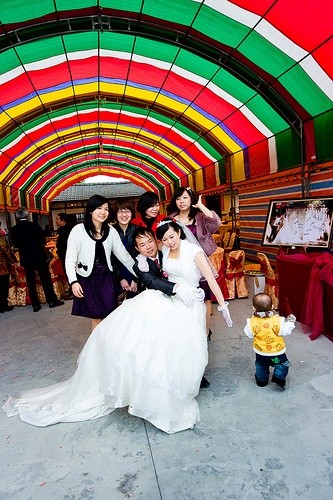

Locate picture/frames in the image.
[261,196,333,251]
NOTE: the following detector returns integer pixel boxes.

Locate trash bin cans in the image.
[243,270,266,298]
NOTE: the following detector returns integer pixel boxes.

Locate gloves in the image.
[197,288,205,304]
[176,284,195,305]
[217,301,233,327]
[136,254,149,272]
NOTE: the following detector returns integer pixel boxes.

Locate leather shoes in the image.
[33,304,41,312]
[64,294,74,300]
[49,300,64,308]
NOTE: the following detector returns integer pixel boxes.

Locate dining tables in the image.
[44,236,58,258]
[211,220,240,253]
[198,246,226,304]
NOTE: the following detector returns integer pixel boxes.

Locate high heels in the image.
[207,328,212,341]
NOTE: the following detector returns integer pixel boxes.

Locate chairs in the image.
[7,252,69,307]
[257,252,279,310]
[222,231,248,299]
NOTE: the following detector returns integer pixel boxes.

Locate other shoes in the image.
[0,306,15,313]
[256,381,268,388]
[200,376,210,389]
[271,382,285,391]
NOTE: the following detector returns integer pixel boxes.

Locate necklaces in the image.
[170,250,177,258]
[179,214,189,224]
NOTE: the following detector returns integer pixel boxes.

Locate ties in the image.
[154,259,161,270]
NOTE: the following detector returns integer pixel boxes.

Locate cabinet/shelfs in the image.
[276,252,333,343]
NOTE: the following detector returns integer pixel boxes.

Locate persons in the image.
[244,293,296,391]
[0,186,234,435]
